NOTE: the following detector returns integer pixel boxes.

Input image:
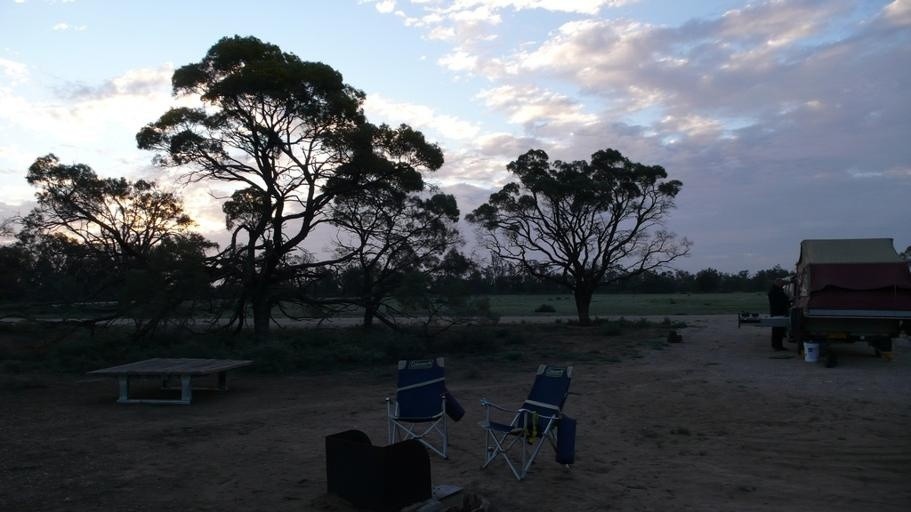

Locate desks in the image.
[88,356,255,405]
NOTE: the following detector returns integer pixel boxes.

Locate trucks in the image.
[783,237,910,360]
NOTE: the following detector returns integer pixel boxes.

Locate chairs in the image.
[325,358,577,511]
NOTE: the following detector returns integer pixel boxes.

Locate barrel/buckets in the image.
[802,341,820,364]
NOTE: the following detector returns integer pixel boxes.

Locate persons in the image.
[769,273,792,352]
